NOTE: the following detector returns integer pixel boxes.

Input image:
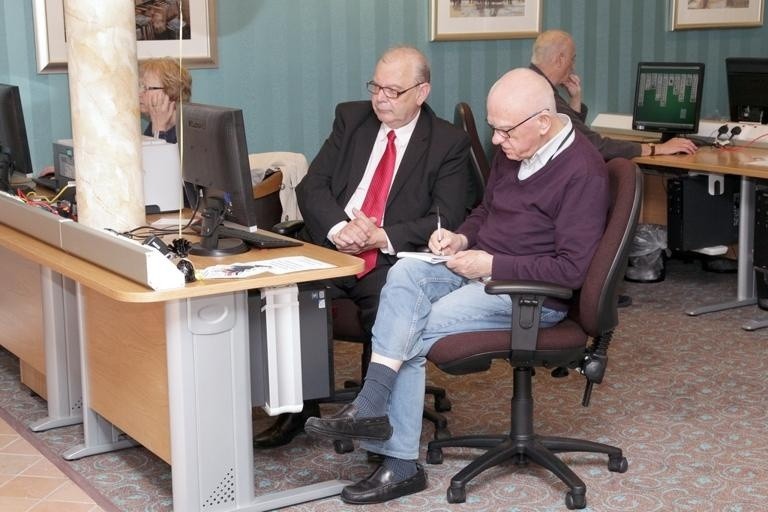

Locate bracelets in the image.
[648,142,656,156]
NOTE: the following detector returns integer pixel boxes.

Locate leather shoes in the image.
[305,404,392,440]
[253,401,321,450]
[342,465,429,504]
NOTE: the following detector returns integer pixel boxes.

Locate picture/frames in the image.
[429,0,543,41]
[669,0,764,32]
[31,0,219,76]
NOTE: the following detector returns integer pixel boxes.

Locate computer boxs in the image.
[248,285,334,408]
[666,175,738,251]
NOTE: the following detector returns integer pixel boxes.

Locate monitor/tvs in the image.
[0,82,34,196]
[726,57,768,124]
[631,61,705,142]
[175,101,257,257]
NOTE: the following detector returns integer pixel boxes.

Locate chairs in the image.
[271,100,452,454]
[428,156,644,511]
[454,102,491,213]
[246,152,303,235]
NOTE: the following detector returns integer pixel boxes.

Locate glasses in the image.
[366,81,425,99]
[486,108,549,138]
[138,84,165,94]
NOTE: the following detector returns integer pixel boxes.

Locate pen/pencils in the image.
[437,206,442,255]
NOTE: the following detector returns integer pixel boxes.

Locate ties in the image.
[351,131,395,278]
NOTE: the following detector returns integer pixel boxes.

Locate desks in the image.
[586,126,768,330]
[0,181,365,512]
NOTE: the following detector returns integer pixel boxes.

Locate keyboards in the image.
[189,225,303,249]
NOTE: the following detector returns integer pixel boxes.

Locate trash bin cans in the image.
[624,223,668,284]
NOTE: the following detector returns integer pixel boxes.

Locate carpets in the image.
[0,265,766,511]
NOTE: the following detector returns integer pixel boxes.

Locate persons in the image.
[34,56,192,181]
[527,29,699,163]
[249,46,485,451]
[303,67,611,505]
